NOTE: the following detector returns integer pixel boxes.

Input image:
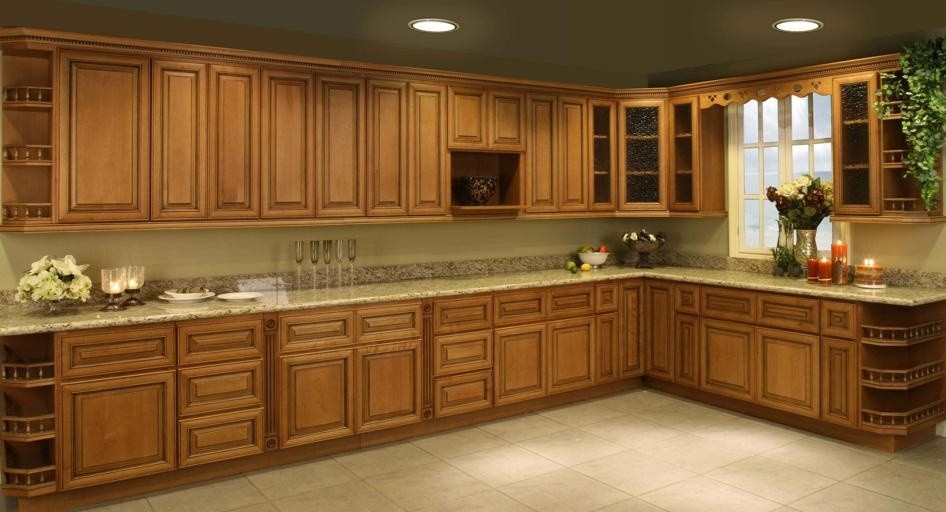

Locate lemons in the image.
[571,267,577,273]
[580,263,591,271]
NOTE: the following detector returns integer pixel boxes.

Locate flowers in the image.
[621,224,668,244]
[764,170,837,233]
[12,251,95,306]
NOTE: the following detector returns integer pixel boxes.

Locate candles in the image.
[853,255,886,286]
[107,281,122,292]
[817,256,834,288]
[805,256,818,284]
[126,277,140,289]
[831,238,851,284]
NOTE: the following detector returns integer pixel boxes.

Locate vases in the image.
[40,297,73,314]
[789,223,821,278]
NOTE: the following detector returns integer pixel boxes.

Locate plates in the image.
[217,292,263,303]
[158,287,216,303]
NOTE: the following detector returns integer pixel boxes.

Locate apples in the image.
[597,246,608,252]
[577,245,594,253]
[565,260,576,271]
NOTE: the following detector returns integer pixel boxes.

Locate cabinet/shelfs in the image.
[175,316,267,466]
[524,91,592,216]
[59,321,176,496]
[820,299,859,432]
[271,299,425,453]
[56,45,153,224]
[446,83,528,219]
[701,286,822,420]
[586,96,618,216]
[664,92,726,215]
[0,38,58,232]
[645,277,701,391]
[860,298,946,441]
[490,279,593,407]
[876,64,944,219]
[596,275,647,385]
[618,96,665,215]
[260,66,368,219]
[830,71,885,219]
[429,290,495,425]
[366,76,448,220]
[0,333,62,498]
[149,56,262,222]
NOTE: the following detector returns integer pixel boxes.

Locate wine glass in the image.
[99,262,148,312]
[295,238,357,292]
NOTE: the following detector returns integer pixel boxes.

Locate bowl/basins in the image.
[577,251,609,269]
[458,175,499,205]
